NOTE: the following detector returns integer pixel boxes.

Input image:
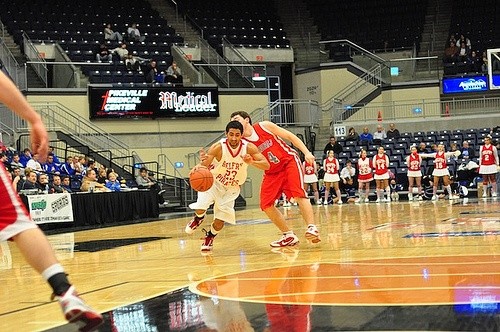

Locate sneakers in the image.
[304,224,321,243]
[200,228,214,251]
[270,231,300,247]
[50,284,104,332]
[185,213,205,234]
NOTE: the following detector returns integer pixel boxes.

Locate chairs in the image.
[305,0,428,61]
[320,125,500,192]
[0,0,291,84]
[444,0,500,75]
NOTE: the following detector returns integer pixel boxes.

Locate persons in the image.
[95,22,183,86]
[0,67,104,325]
[0,142,169,205]
[477,126,500,197]
[323,136,342,154]
[406,141,480,200]
[201,111,321,247]
[185,121,270,252]
[347,124,400,141]
[442,33,498,77]
[301,145,403,205]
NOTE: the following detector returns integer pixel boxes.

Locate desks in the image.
[19,188,158,230]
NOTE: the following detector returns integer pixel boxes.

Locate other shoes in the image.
[315,201,321,205]
[324,201,328,205]
[354,198,361,203]
[492,193,497,196]
[431,196,437,201]
[293,202,297,206]
[385,199,391,202]
[365,198,369,203]
[408,193,413,201]
[419,196,422,201]
[283,201,290,207]
[482,193,487,197]
[338,200,343,204]
[376,199,380,203]
[449,195,453,200]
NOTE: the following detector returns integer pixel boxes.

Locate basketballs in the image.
[189,168,214,193]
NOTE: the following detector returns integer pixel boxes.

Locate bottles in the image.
[45,184,49,194]
